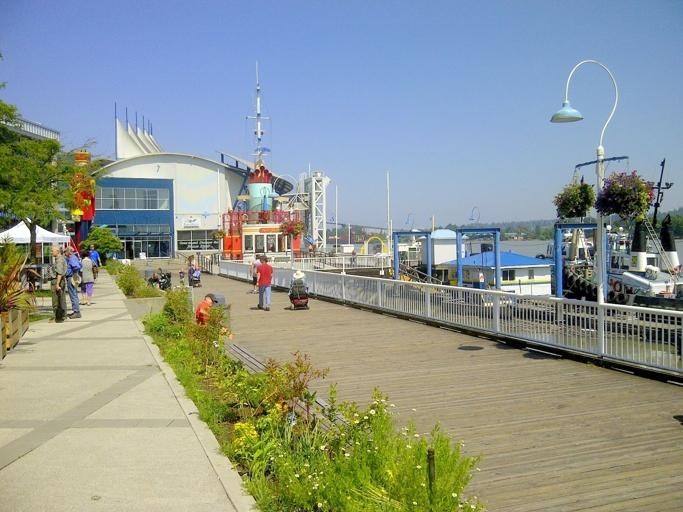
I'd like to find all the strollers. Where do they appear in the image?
[189,271,200,288]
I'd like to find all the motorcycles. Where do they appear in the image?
[155,267,172,290]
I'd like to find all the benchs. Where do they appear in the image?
[219,341,371,459]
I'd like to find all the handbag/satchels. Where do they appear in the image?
[55,290,64,320]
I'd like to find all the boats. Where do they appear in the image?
[543,156,683,346]
[440,290,515,320]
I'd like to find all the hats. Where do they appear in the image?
[71,274,82,288]
[293,270,305,279]
[206,294,218,303]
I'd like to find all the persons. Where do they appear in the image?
[255,256,273,311]
[63,246,82,320]
[349,250,357,267]
[78,250,96,306]
[250,254,261,294]
[196,293,218,325]
[187,263,195,281]
[87,243,102,280]
[48,245,68,323]
[192,267,200,279]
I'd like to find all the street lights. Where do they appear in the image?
[547,59,619,360]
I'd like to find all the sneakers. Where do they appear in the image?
[69,311,81,319]
[49,319,63,324]
[257,304,263,309]
[266,307,270,311]
[67,311,76,317]
